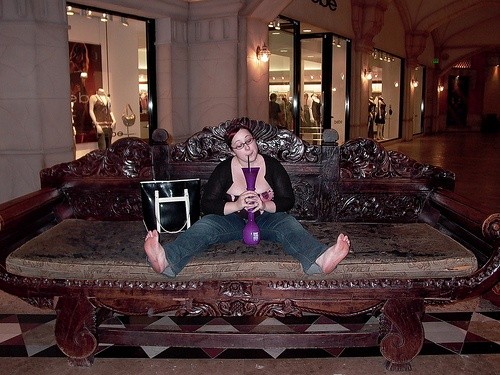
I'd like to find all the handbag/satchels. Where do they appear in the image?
[122,104,136,126]
[139,178,202,233]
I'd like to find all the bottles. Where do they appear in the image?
[242,167,260,246]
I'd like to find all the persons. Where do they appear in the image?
[368,96,386,140]
[89,88,116,151]
[269,94,281,127]
[144,124,350,278]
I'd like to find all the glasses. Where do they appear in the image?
[232,135,254,150]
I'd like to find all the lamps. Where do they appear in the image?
[67,6,75,16]
[333,36,342,48]
[439,82,445,92]
[412,77,419,88]
[362,68,373,80]
[108,15,114,23]
[371,48,395,63]
[268,19,281,30]
[256,43,272,63]
[100,14,107,22]
[121,17,129,27]
[87,11,93,19]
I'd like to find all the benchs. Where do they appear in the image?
[0,114,500,373]
